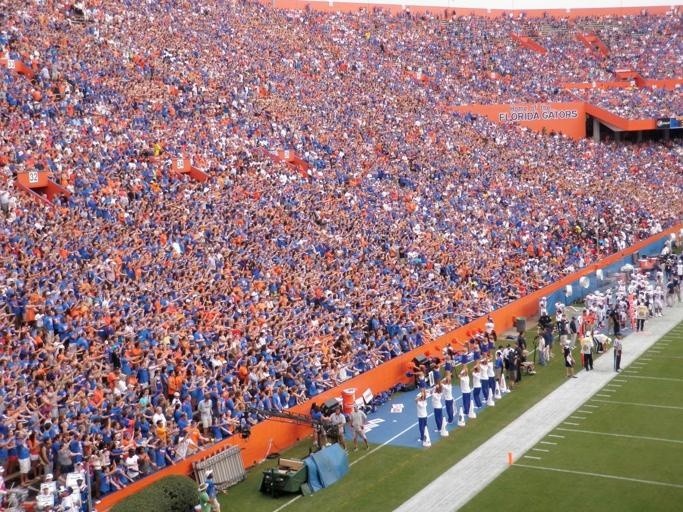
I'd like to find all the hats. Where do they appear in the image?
[506,344,511,348]
[206,469,213,477]
[616,333,623,335]
[197,482,209,492]
[335,405,342,409]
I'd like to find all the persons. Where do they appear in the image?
[0,0,682,512]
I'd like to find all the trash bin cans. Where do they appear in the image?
[320,388,355,415]
[515,318,525,332]
[412,354,426,366]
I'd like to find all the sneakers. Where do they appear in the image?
[344,448,348,451]
[366,446,370,451]
[463,413,469,416]
[648,313,663,318]
[615,368,621,372]
[482,397,487,404]
[354,448,358,452]
[568,374,578,378]
[314,441,318,445]
[474,406,481,408]
[433,429,440,432]
[326,442,332,446]
[621,327,628,330]
[586,366,593,370]
[418,438,422,442]
